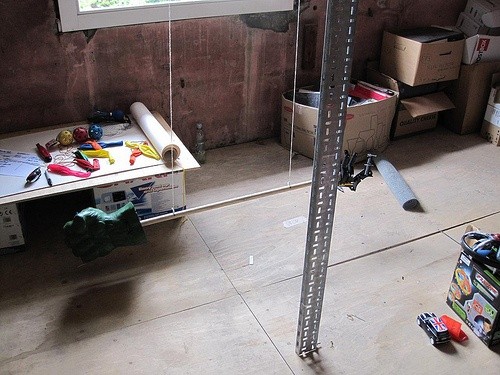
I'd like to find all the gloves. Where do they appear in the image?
[63,202,147,263]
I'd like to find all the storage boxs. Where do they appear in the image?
[0,204,26,248]
[92,172,188,222]
[446,233,500,348]
[277,0,500,163]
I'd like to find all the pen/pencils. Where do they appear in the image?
[45,170,52,186]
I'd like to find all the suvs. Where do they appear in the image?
[416,312,451,345]
